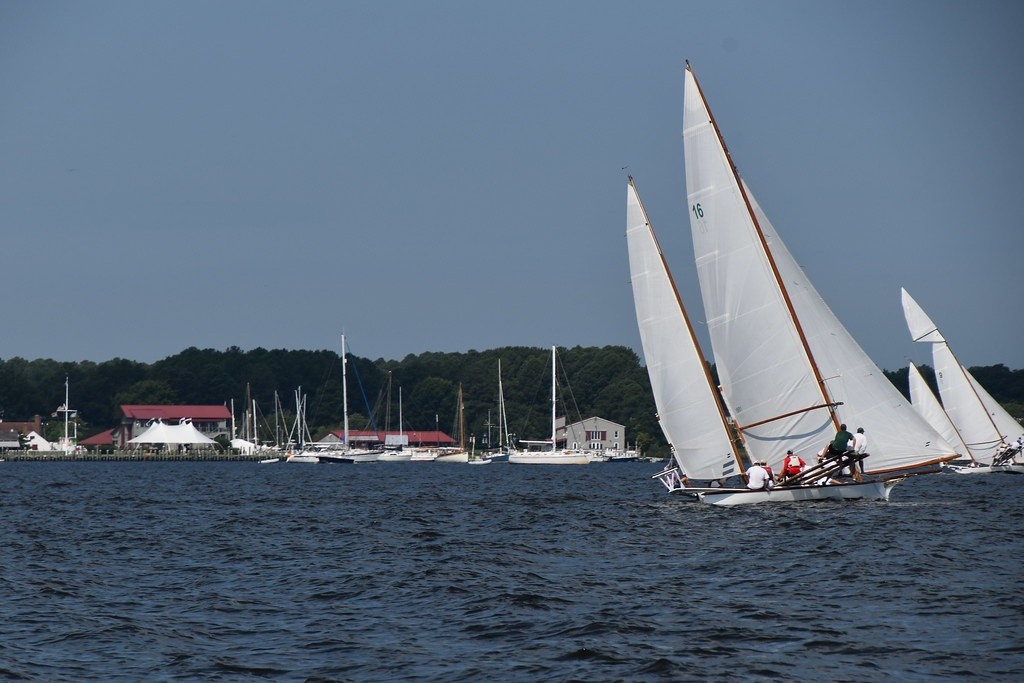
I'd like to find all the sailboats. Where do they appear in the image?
[625,57,964,508]
[231,328,665,465]
[898,286,1024,475]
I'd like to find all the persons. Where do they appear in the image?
[740,460,774,492]
[992,437,1022,461]
[777,450,806,480]
[707,479,723,488]
[849,428,868,473]
[817,440,841,485]
[830,424,856,458]
[664,465,688,485]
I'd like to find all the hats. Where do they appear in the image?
[787,450,793,454]
[761,460,767,463]
[753,460,760,464]
[857,428,865,433]
